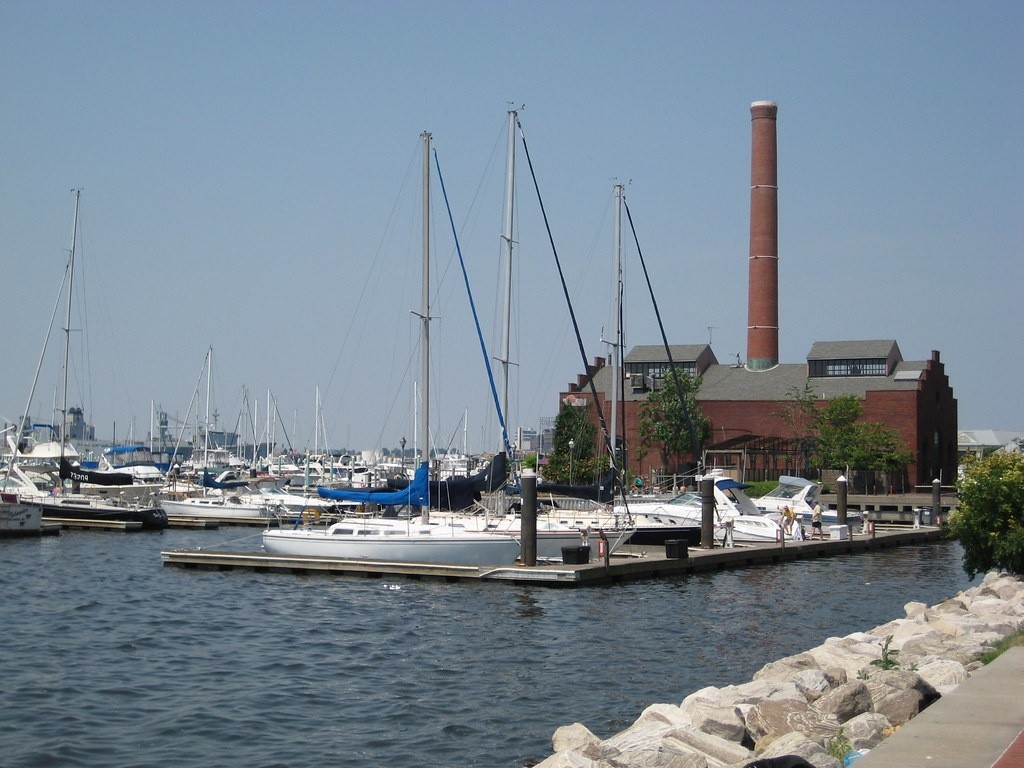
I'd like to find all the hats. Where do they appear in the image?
[811,500,818,504]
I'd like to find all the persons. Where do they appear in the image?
[779,505,796,535]
[632,475,686,495]
[809,500,824,540]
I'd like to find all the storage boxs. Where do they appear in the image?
[561,546,590,564]
[664,539,690,558]
[828,524,847,540]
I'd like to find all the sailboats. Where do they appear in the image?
[0,106,852,570]
